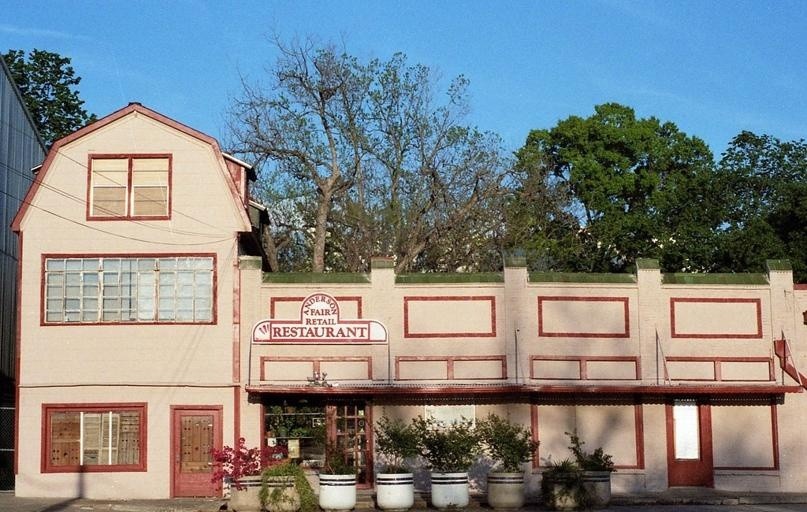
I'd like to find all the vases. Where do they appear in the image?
[230,475,262,512]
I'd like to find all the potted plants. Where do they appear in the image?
[473,410,540,511]
[310,418,362,512]
[370,415,433,511]
[563,429,618,510]
[260,412,323,511]
[542,457,583,510]
[413,414,493,510]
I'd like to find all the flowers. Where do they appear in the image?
[209,438,290,498]
